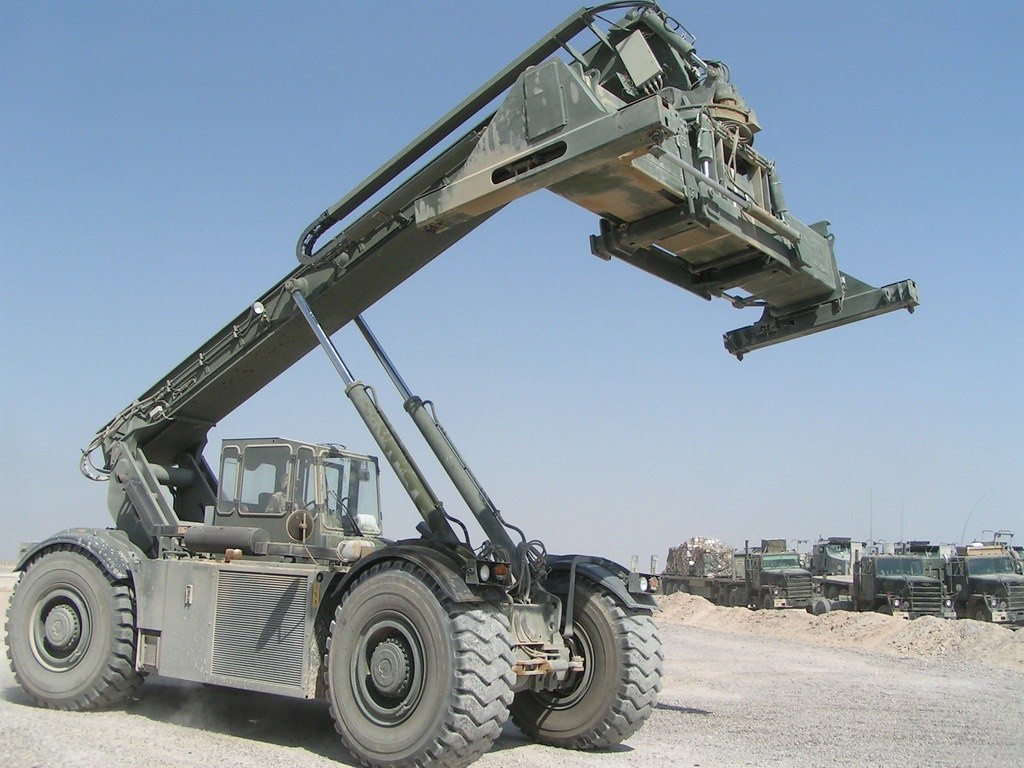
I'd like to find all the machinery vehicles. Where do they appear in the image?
[2,0,920,768]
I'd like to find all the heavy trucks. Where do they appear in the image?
[659,530,1024,631]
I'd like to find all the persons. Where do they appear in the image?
[265,473,309,512]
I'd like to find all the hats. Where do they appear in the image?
[280,473,300,490]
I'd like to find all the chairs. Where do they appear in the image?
[259,492,275,504]
